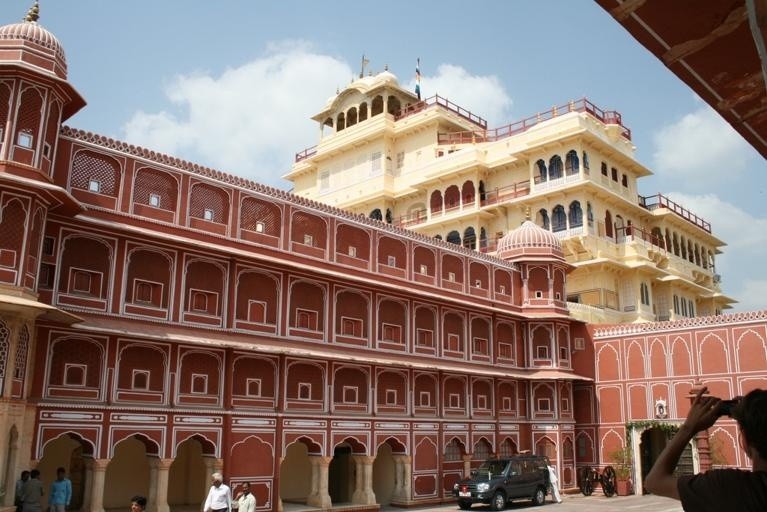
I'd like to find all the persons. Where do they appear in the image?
[21,469,45,512]
[13,470,30,512]
[201,472,232,512]
[231,481,256,512]
[642,384,767,512]
[130,495,147,512]
[47,466,73,512]
[546,464,562,504]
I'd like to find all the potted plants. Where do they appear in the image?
[608,443,634,497]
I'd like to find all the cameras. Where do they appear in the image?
[718,400,737,415]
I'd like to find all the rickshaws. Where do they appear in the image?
[577,461,617,498]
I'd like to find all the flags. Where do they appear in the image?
[361,55,370,68]
[414,59,420,94]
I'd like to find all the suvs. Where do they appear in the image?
[451,453,550,511]
